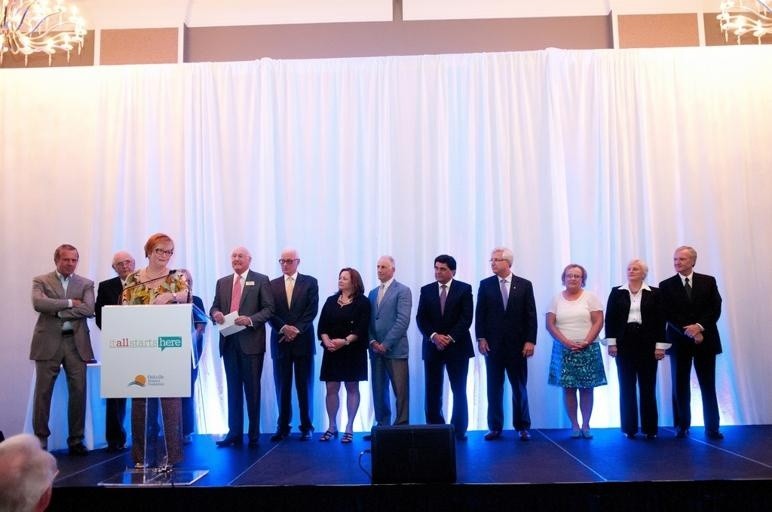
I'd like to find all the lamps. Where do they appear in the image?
[1,4,88,73]
[718,2,772,47]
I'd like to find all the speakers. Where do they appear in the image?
[372,426,456,484]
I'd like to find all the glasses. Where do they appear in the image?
[279,259,298,264]
[155,248,173,257]
[489,257,507,262]
[117,260,132,266]
[565,274,582,278]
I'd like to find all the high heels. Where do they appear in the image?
[42,444,48,451]
[320,429,338,442]
[341,430,353,444]
[645,432,657,442]
[625,432,635,440]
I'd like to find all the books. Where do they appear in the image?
[214,310,246,337]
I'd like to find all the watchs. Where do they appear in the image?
[172,292,178,303]
[344,337,349,345]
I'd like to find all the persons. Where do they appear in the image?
[209,245,276,450]
[604,258,672,439]
[317,267,371,444]
[173,269,208,445]
[29,244,95,457]
[121,233,188,470]
[416,254,475,441]
[659,246,724,440]
[545,264,608,439]
[94,250,135,453]
[0,434,60,512]
[268,246,319,441]
[475,246,537,440]
[362,254,412,441]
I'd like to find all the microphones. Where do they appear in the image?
[119,269,177,301]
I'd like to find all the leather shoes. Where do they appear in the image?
[216,437,244,446]
[571,425,582,438]
[104,444,124,456]
[364,434,377,442]
[67,442,93,458]
[300,428,314,441]
[706,430,723,440]
[272,429,289,441]
[584,425,593,439]
[674,426,690,438]
[455,433,468,442]
[484,429,502,441]
[247,437,260,449]
[518,429,532,441]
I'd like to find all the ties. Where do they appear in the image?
[230,277,242,313]
[440,285,447,316]
[286,277,293,311]
[500,279,508,310]
[684,278,692,303]
[378,284,385,304]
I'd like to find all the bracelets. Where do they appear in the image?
[583,340,589,346]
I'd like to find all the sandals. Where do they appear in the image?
[146,434,160,444]
[182,434,193,444]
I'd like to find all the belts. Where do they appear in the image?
[62,329,74,338]
